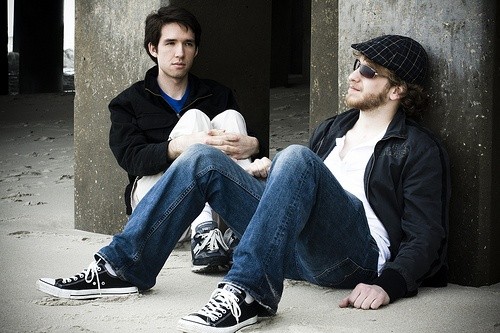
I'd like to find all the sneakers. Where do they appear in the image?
[224,227,239,265]
[178,283,258,333]
[190,221,229,274]
[36,259,138,300]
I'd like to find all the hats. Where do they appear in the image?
[350,34,428,86]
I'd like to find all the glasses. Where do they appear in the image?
[352,59,393,84]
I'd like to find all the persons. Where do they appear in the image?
[36,35,453,333]
[108,6,264,273]
